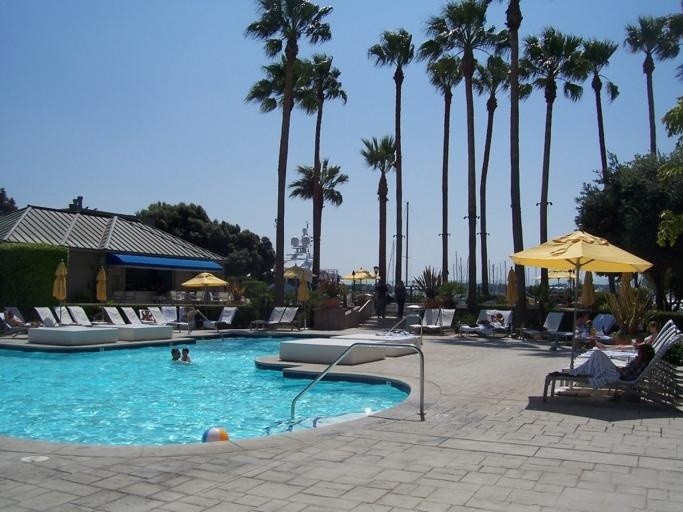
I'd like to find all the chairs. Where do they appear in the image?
[249,306,306,333]
[200,306,238,331]
[1,288,191,340]
[397,284,682,408]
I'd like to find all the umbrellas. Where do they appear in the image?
[534,269,577,287]
[181,269,229,315]
[95,264,106,320]
[298,270,311,330]
[343,267,377,293]
[508,224,653,396]
[52,259,69,325]
[505,267,518,311]
[284,263,310,293]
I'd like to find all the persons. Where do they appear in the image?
[479,314,504,332]
[171,348,180,359]
[174,306,197,323]
[3,308,41,326]
[568,310,658,381]
[179,349,191,363]
[140,310,154,321]
[394,279,408,319]
[377,277,389,318]
